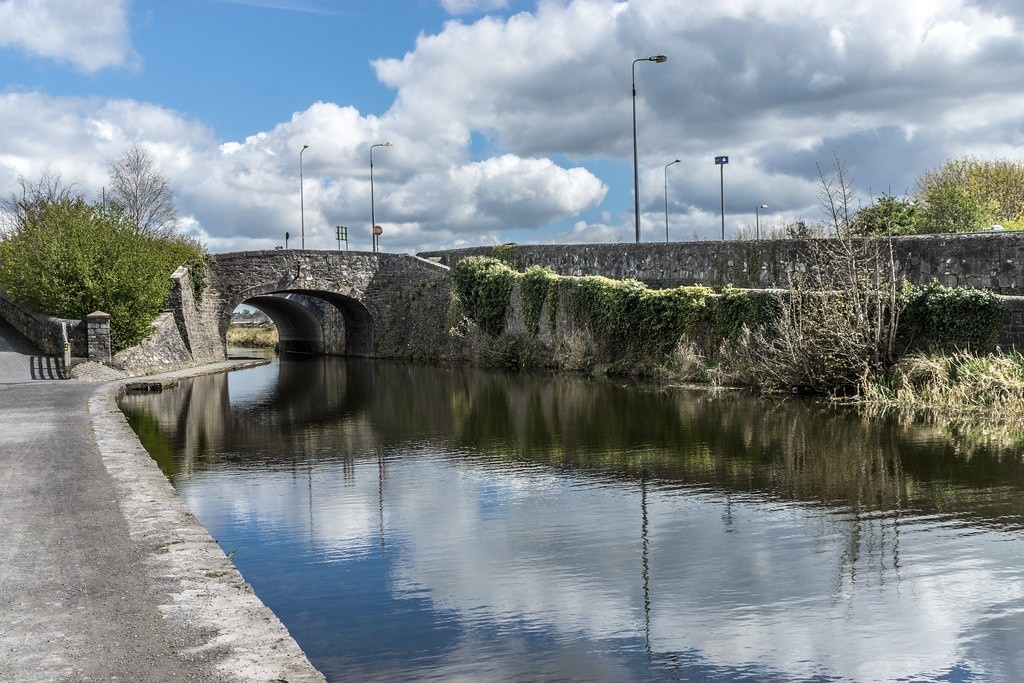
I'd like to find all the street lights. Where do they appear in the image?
[665,159,682,242]
[756,205,768,240]
[632,55,667,242]
[300,145,308,249]
[370,142,393,251]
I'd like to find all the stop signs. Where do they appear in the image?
[374,226,382,235]
